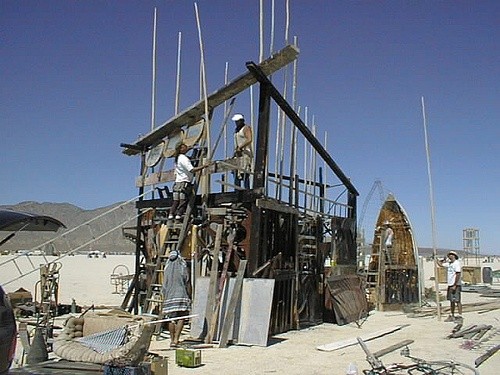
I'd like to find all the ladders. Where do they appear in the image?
[143,146,206,341]
[35,276,54,342]
[216,153,353,218]
[121,257,145,314]
[356,228,365,297]
[0,189,154,286]
[365,227,381,311]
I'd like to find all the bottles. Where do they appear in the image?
[72,301,76,312]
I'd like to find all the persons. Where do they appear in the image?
[166,141,211,224]
[381,220,394,265]
[162,249,192,348]
[435,250,463,322]
[231,114,255,191]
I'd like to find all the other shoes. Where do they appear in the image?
[167,218,174,224]
[170,341,187,348]
[444,315,455,322]
[455,315,462,320]
[174,218,182,224]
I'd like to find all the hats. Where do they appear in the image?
[447,251,459,259]
[231,114,244,120]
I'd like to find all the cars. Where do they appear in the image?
[0,208,67,375]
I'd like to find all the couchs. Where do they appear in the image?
[53,317,157,366]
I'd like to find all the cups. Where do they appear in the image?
[331,260,336,266]
